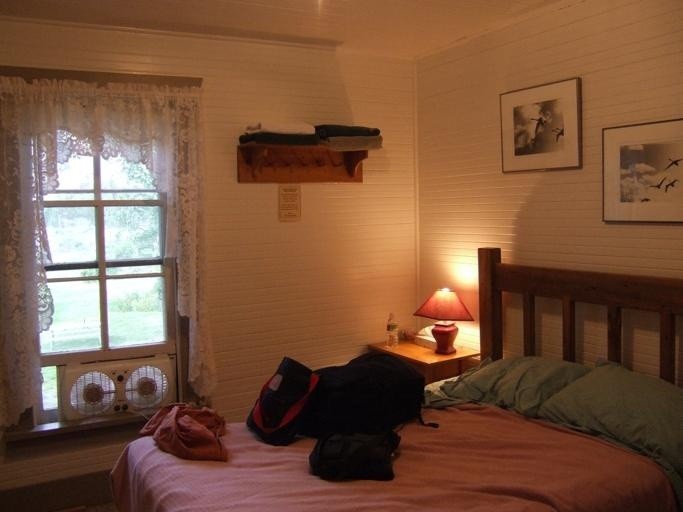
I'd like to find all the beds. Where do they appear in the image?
[127,245,681,512]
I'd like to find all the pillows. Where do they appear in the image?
[423,350,681,492]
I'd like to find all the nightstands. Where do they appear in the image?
[368,338,481,394]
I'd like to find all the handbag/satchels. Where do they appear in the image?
[297,351,426,440]
[245,356,320,447]
[308,429,401,482]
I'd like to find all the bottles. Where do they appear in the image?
[387,312,399,352]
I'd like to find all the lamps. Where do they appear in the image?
[412,286,474,355]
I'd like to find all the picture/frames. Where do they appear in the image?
[498,71,682,225]
